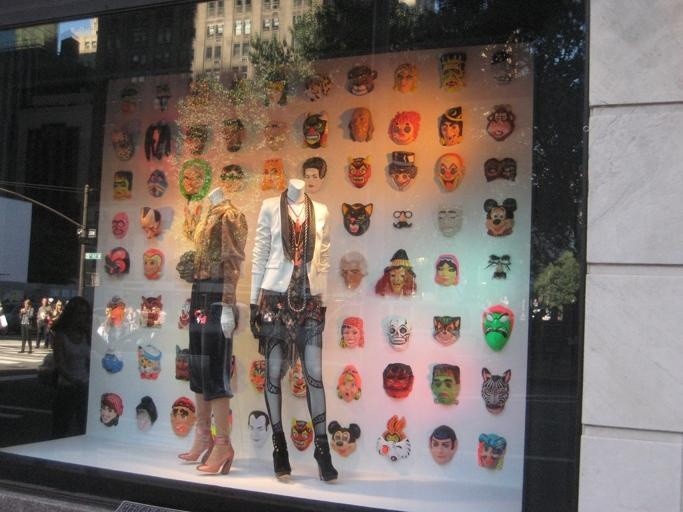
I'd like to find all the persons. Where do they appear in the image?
[0,298,64,353]
[249,180,339,482]
[49,296,91,439]
[179,187,247,474]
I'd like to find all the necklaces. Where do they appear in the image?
[286,192,309,313]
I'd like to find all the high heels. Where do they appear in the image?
[314,435,338,481]
[272,432,291,480]
[178,429,234,474]
